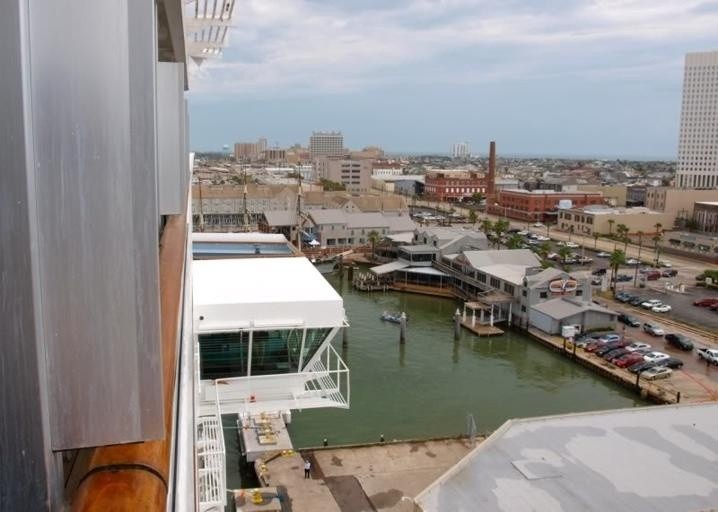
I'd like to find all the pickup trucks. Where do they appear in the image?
[696,347,718,366]
[663,332,694,350]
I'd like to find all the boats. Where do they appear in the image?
[381,312,409,325]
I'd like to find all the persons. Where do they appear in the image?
[303,459,311,479]
[622,324,626,335]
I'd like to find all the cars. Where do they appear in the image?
[641,300,663,310]
[613,352,642,368]
[612,273,634,282]
[591,276,602,285]
[657,358,685,369]
[596,251,611,258]
[709,302,718,311]
[622,314,641,327]
[486,226,593,265]
[575,333,634,362]
[592,267,606,275]
[624,341,652,352]
[641,366,672,380]
[643,351,670,364]
[614,290,646,307]
[693,297,718,306]
[412,209,465,225]
[624,258,677,280]
[618,312,625,322]
[651,304,672,313]
[534,222,544,227]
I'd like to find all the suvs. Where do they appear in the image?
[643,322,663,336]
